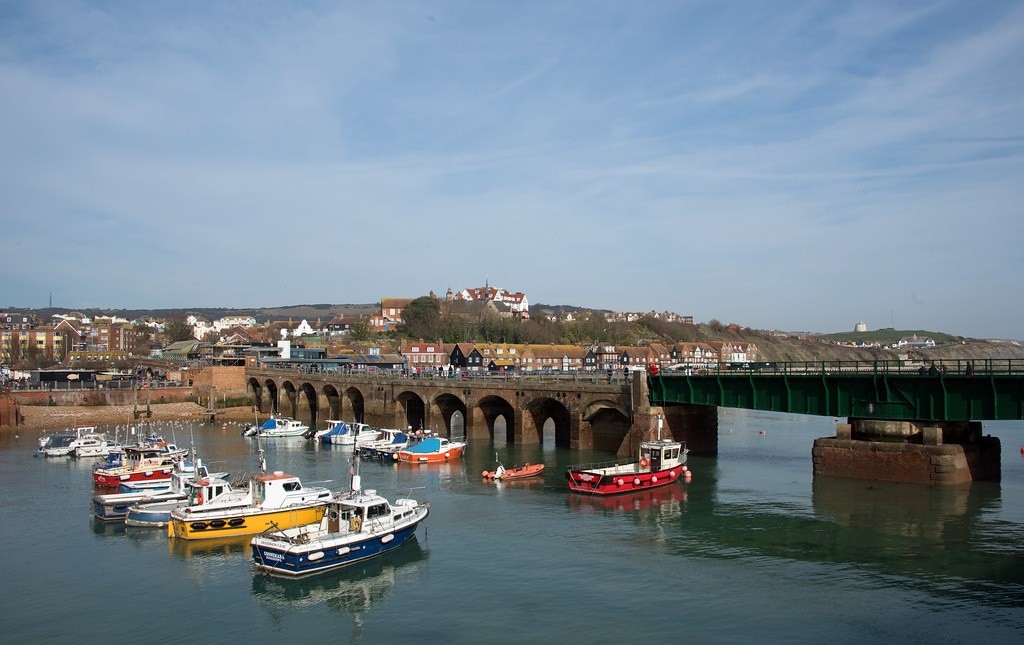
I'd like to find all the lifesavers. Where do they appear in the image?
[198,493,203,505]
[351,518,361,531]
[153,442,168,446]
[200,482,209,486]
[640,457,649,469]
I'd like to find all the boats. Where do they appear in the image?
[118,423,231,493]
[314,420,353,443]
[483,452,546,481]
[166,406,342,540]
[355,426,439,460]
[125,459,250,527]
[566,413,689,495]
[330,422,382,445]
[37,425,189,487]
[396,432,469,464]
[242,399,310,436]
[251,430,429,575]
[92,472,196,522]
